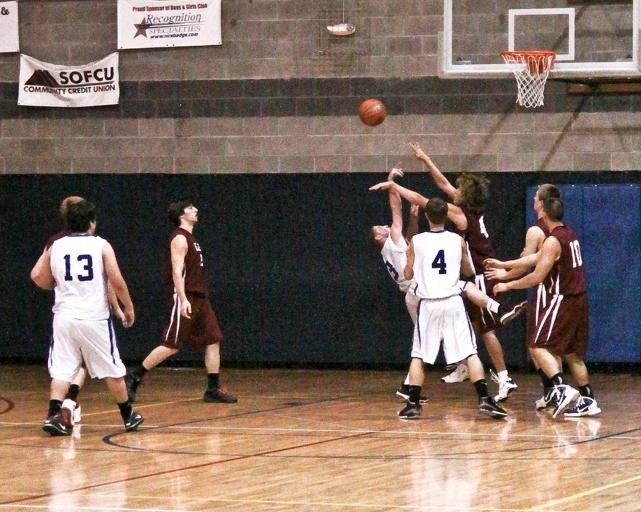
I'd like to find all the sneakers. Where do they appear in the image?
[440,363,470,384]
[204,384,237,403]
[395,402,420,419]
[496,300,528,326]
[42,404,143,436]
[395,381,429,403]
[123,368,145,402]
[478,395,508,419]
[489,369,519,403]
[535,384,600,419]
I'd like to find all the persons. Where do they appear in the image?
[121,194,238,404]
[482,196,604,420]
[40,194,129,427]
[30,200,145,436]
[397,196,512,422]
[482,182,564,410]
[366,139,520,406]
[359,160,529,406]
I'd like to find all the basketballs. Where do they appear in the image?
[358,97,387,126]
